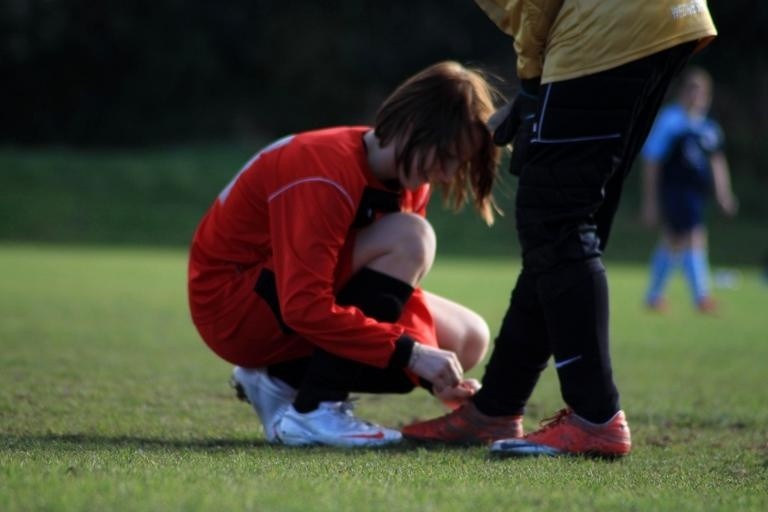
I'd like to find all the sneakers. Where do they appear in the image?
[229,366,406,449]
[492,409,632,458]
[402,400,525,446]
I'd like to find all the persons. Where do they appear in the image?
[401,0,718,458]
[638,71,736,315]
[188,61,515,449]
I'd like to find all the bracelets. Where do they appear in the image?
[409,343,422,369]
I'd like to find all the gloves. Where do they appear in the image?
[487,74,542,176]
